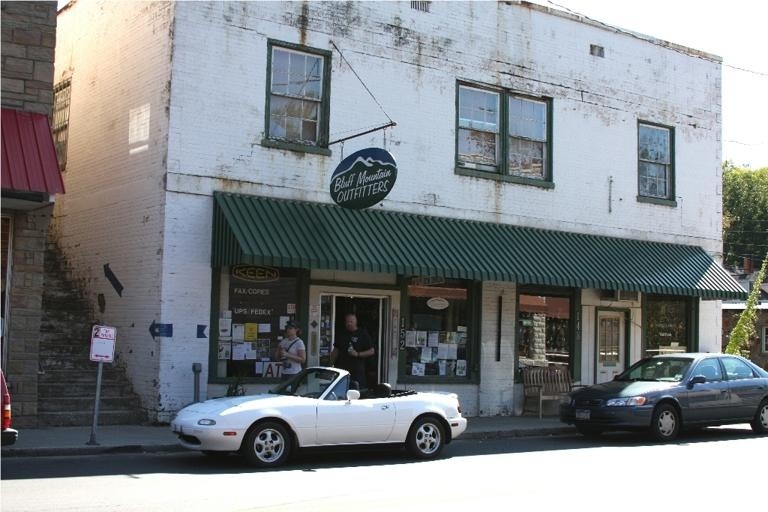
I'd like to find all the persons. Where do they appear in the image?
[330,311,376,390]
[272,320,305,386]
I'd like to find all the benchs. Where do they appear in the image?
[520,366,590,419]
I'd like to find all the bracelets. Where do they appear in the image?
[286,352,289,357]
[357,350,360,359]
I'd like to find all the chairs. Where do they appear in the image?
[349,381,360,391]
[652,361,671,378]
[729,367,751,379]
[700,366,717,379]
[365,383,392,398]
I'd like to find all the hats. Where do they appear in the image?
[286,320,302,329]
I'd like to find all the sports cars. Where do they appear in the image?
[169,364,468,467]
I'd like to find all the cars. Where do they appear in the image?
[558,353,768,439]
